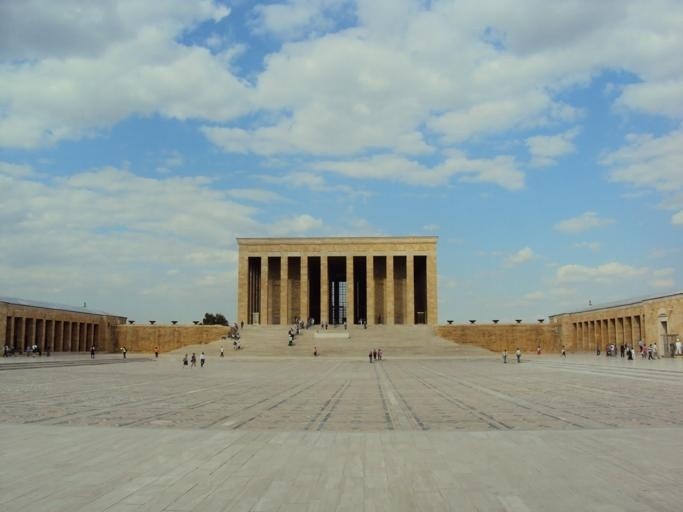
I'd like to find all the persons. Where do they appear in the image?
[560,345,566,359]
[319,321,324,331]
[89,345,95,359]
[367,348,382,364]
[595,339,660,361]
[668,341,675,358]
[675,338,682,355]
[343,322,347,330]
[312,347,317,356]
[228,320,244,351]
[287,315,315,346]
[190,352,196,368]
[219,346,223,357]
[536,345,541,355]
[325,322,328,329]
[153,347,159,358]
[514,347,521,363]
[501,349,507,364]
[181,353,188,368]
[0,343,51,358]
[119,346,126,360]
[198,352,206,367]
